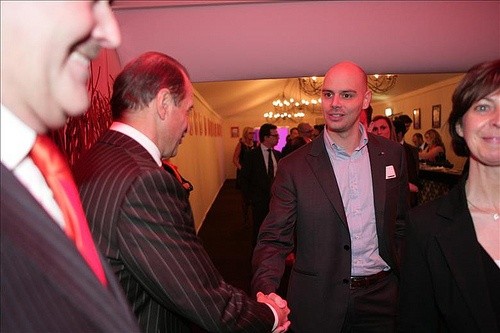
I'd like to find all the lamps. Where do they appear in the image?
[264,73,397,123]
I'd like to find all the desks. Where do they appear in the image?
[418,165,461,205]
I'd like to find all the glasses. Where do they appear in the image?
[270,134,279,138]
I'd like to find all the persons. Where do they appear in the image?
[242,124,285,247]
[398,58,500,333]
[370,114,454,206]
[233,127,256,189]
[0,1,144,333]
[72,53,288,333]
[282,122,325,157]
[253,60,413,333]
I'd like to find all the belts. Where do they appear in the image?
[349,269,394,289]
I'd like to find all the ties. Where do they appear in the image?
[267,149,274,178]
[29,137,106,285]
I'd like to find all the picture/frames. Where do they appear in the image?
[432,104,441,128]
[413,108,421,129]
